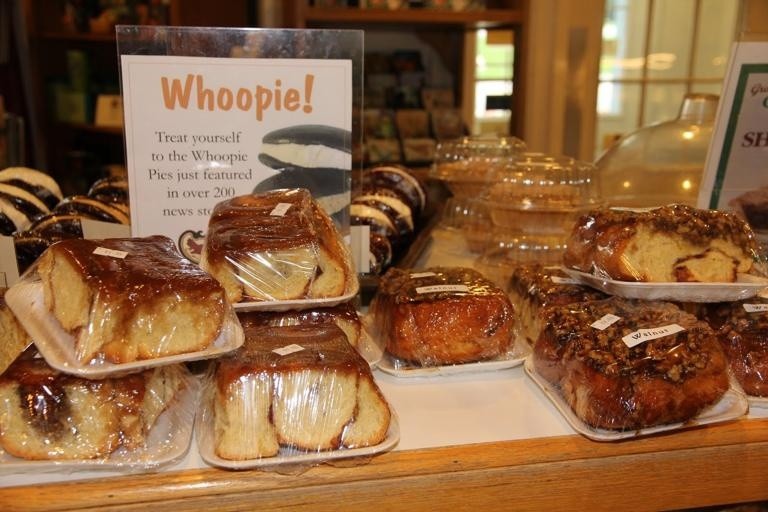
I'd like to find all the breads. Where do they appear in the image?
[0,152,435,470]
[429,150,768,443]
[364,262,522,376]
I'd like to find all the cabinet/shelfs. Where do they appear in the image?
[23,1,181,197]
[285,1,531,177]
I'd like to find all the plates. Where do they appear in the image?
[1,266,767,474]
